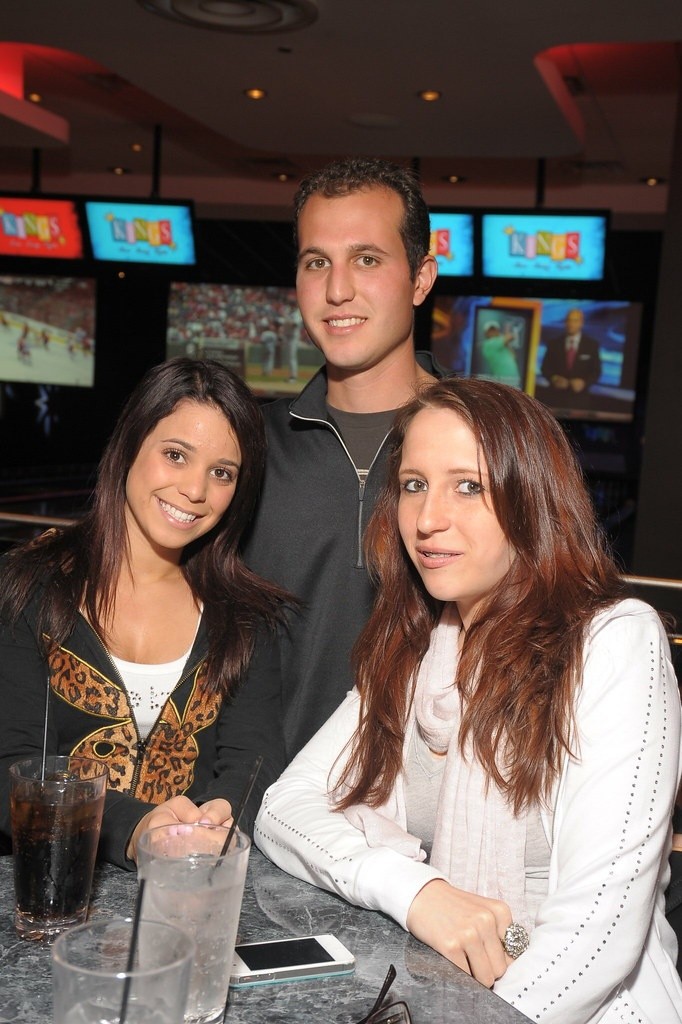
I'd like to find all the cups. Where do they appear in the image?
[135,823,252,1024]
[51,918,194,1024]
[8,755,111,937]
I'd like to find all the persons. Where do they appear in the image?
[540,309,602,393]
[252,378,682,1022]
[481,318,519,388]
[238,158,455,765]
[0,283,95,362]
[0,354,313,873]
[167,283,313,382]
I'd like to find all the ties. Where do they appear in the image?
[567,337,576,368]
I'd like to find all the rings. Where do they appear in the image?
[500,921,530,959]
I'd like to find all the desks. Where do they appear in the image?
[0,842,536,1024]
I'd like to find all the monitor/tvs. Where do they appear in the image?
[0,191,648,426]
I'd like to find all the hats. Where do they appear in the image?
[484,321,498,335]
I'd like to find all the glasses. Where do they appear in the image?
[355,964,411,1024]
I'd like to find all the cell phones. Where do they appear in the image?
[230,933,355,983]
